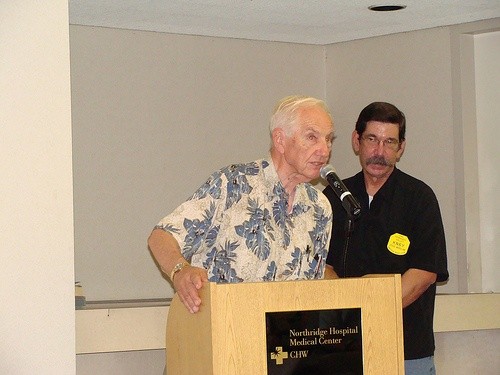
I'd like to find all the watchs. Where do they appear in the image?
[170,262,190,281]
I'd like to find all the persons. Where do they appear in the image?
[323,101,450,375]
[147,94,338,375]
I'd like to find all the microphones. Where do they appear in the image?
[320,164,361,218]
